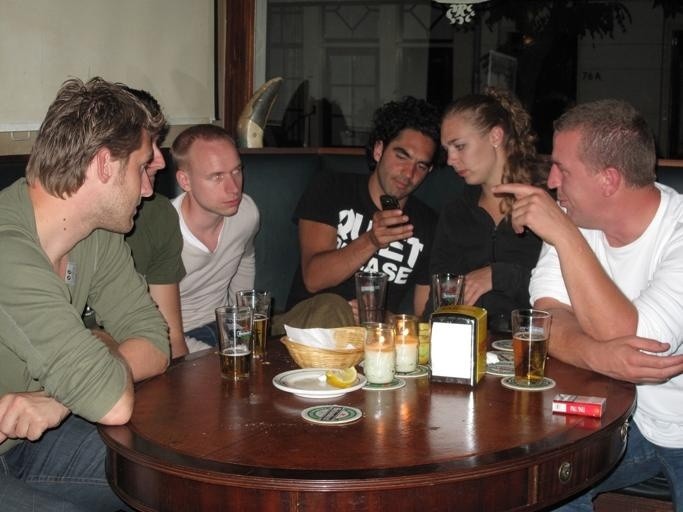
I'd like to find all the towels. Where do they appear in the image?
[284,322,357,350]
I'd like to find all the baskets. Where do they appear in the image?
[281,325,367,369]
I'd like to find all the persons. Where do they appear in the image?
[0,78,170,462]
[270,95,449,341]
[168,124,260,348]
[490,99,683,512]
[81,82,189,360]
[423,83,539,329]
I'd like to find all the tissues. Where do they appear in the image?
[427,304,488,386]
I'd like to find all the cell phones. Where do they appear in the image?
[381,193,402,229]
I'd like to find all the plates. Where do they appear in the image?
[273,367,366,400]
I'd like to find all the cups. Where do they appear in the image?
[237,288,272,359]
[364,322,397,385]
[511,309,550,387]
[355,270,390,326]
[432,273,465,309]
[216,305,255,382]
[392,315,419,373]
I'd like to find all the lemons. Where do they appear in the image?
[326,366,357,386]
